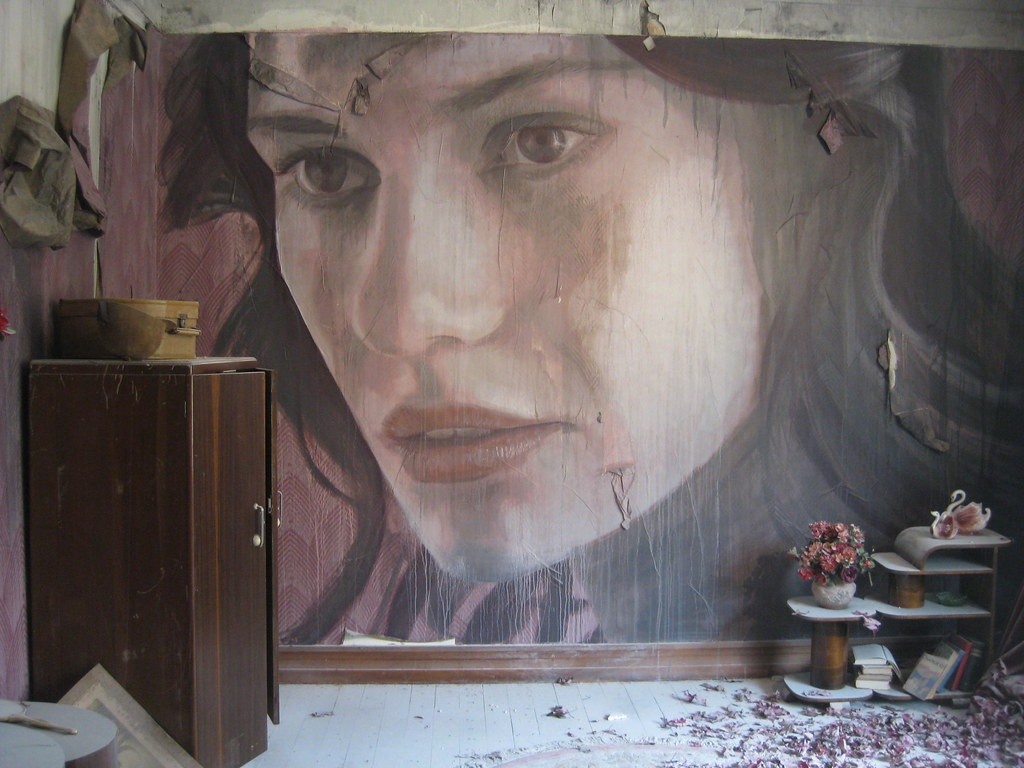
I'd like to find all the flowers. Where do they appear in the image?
[786,520,876,587]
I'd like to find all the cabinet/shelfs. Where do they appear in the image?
[784,528,1013,712]
[17,353,281,768]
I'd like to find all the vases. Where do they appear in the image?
[811,581,857,610]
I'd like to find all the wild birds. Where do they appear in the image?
[930,489,992,540]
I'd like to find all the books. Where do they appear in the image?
[903,635,986,702]
[850,644,905,690]
